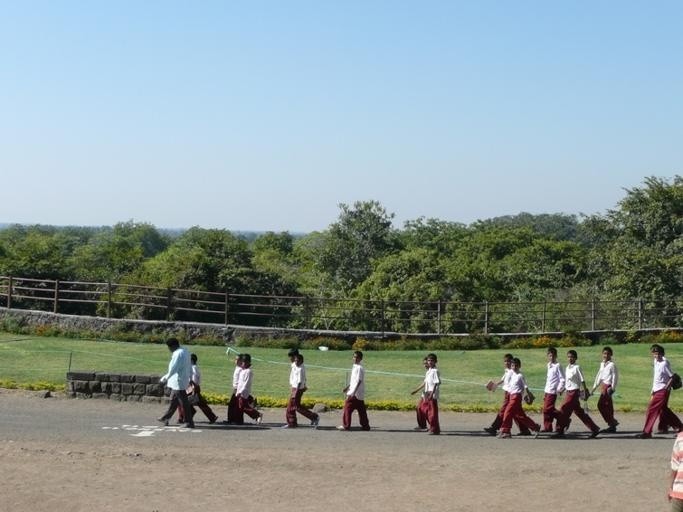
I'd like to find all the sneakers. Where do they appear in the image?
[588,429,616,437]
[517,424,541,438]
[635,433,651,439]
[428,430,440,434]
[183,408,196,421]
[414,426,428,431]
[336,426,346,431]
[484,426,511,438]
[223,420,236,425]
[209,416,218,424]
[250,414,263,425]
[176,419,194,428]
[539,430,564,435]
[284,424,297,428]
[157,417,169,425]
[361,426,370,431]
[311,416,320,425]
[655,424,683,434]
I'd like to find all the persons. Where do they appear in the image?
[666,431,683,512]
[556,350,600,439]
[177,354,218,424]
[336,351,370,431]
[425,353,440,434]
[157,338,196,428]
[500,358,541,439]
[288,348,298,368]
[484,354,531,435]
[589,347,619,432]
[235,354,262,425]
[282,354,320,428]
[411,357,429,429]
[539,347,571,433]
[636,346,683,439]
[650,344,672,434]
[222,355,242,425]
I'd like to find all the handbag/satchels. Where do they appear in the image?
[185,384,200,404]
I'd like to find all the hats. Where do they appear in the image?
[242,354,250,361]
[652,347,664,352]
